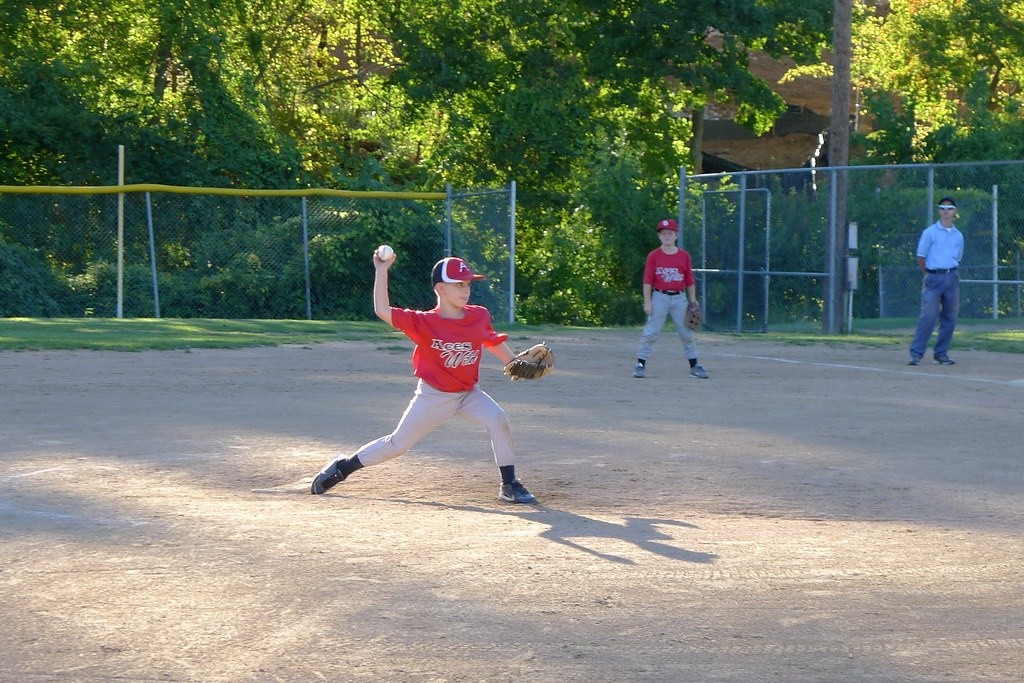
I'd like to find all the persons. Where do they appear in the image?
[310,249,554,504]
[909,196,965,365]
[634,220,709,380]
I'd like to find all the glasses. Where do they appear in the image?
[939,204,955,210]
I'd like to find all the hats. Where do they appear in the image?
[939,196,958,207]
[657,220,678,232]
[431,257,485,287]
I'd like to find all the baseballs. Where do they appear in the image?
[378,245,395,261]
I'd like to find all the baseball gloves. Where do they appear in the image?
[503,344,555,383]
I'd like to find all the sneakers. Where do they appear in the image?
[689,363,709,379]
[906,356,923,365]
[933,355,954,365]
[634,362,646,378]
[310,455,346,494]
[499,479,537,506]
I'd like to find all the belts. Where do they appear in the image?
[927,267,957,274]
[655,288,684,295]
